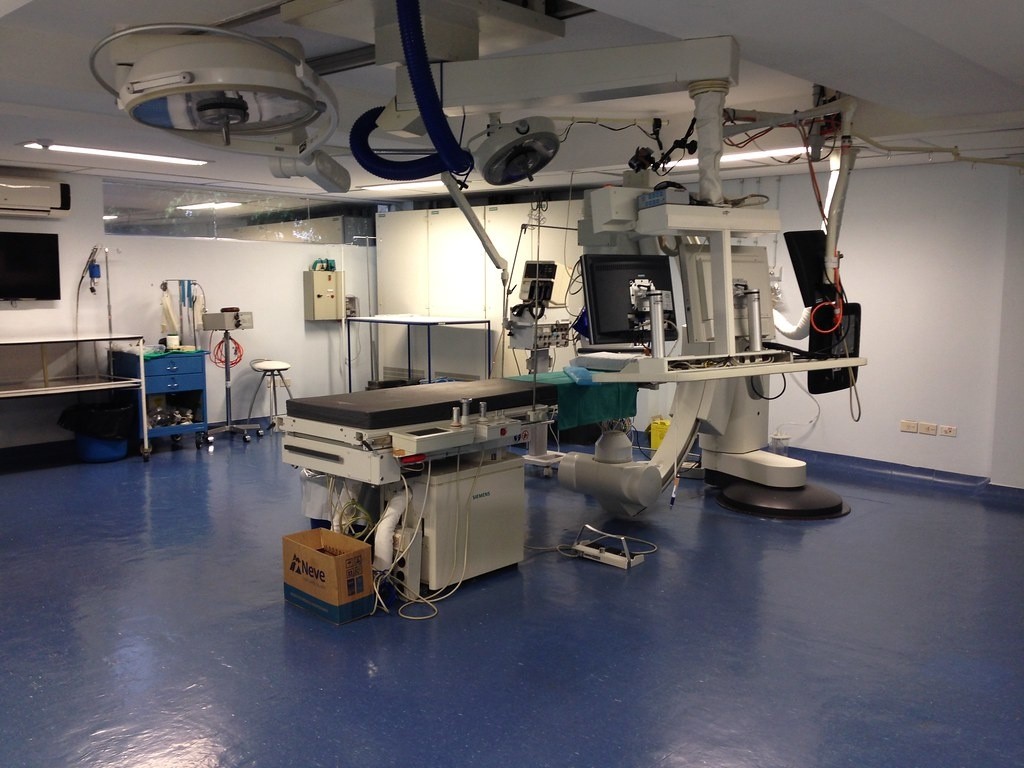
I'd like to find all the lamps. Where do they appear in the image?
[471,114,561,187]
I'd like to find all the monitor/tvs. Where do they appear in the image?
[783,230,843,307]
[580,252,679,346]
[678,243,776,345]
[0,232,60,301]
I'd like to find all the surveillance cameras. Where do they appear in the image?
[628,148,654,170]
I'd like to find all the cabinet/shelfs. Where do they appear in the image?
[344,312,492,393]
[0,331,154,463]
[105,346,211,455]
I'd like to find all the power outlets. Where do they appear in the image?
[939,425,957,437]
[918,422,938,436]
[900,419,918,433]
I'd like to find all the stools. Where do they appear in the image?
[241,360,296,443]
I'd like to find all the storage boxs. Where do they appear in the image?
[54,399,139,464]
[282,527,377,626]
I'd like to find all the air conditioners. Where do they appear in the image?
[0,174,72,220]
